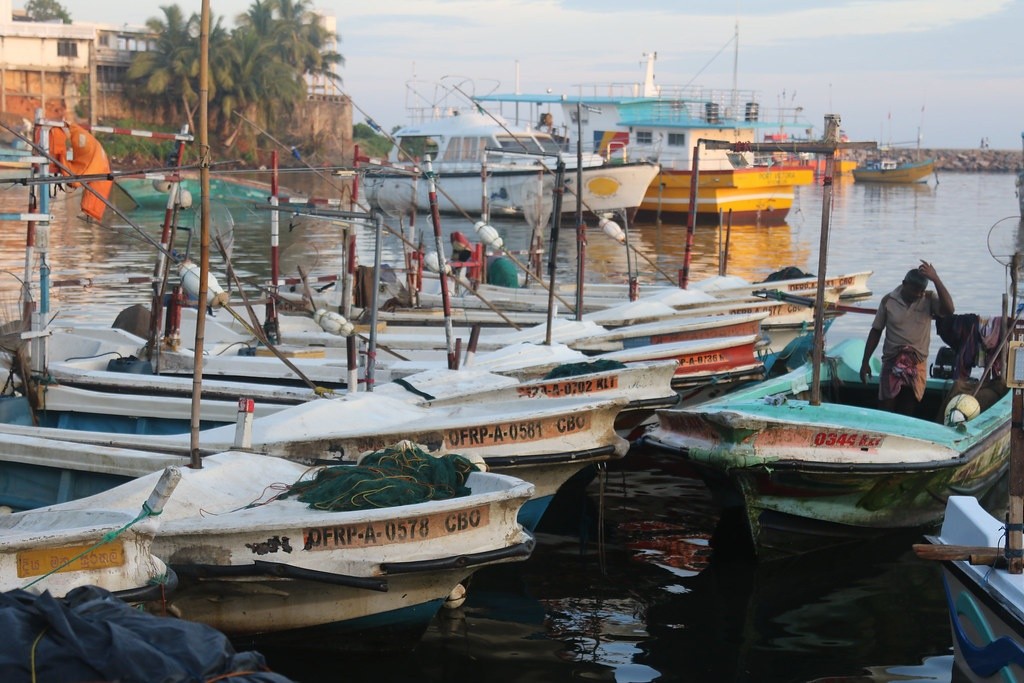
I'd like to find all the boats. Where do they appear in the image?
[853,132,939,184]
[0,142,1024,682]
[354,71,663,233]
[469,50,815,227]
[760,91,830,183]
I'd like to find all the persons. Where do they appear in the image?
[859,259,954,414]
